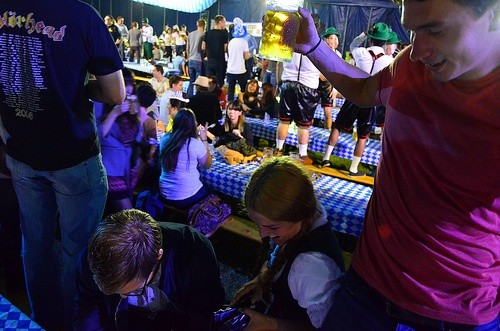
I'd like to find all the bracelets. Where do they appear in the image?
[303,36,322,56]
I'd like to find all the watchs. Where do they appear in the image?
[201,137,207,141]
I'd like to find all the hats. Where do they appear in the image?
[321,27,341,38]
[142,17,149,23]
[166,25,170,31]
[179,31,185,35]
[173,24,179,31]
[192,75,212,87]
[367,23,392,40]
[387,31,401,44]
[231,24,247,38]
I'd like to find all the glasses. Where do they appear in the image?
[124,277,149,296]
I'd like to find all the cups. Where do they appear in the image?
[127,97,139,114]
[259,0,305,63]
[289,152,299,160]
[273,118,278,127]
[208,144,214,161]
[235,85,240,95]
[353,127,358,141]
[263,147,273,160]
[224,85,228,94]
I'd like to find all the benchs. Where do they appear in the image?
[264,154,374,186]
[220,216,352,274]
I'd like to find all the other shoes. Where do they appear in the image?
[348,171,366,178]
[274,149,284,156]
[322,160,331,167]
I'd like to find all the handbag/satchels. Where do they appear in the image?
[185,193,231,235]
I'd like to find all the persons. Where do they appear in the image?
[92,67,143,210]
[103,14,159,64]
[316,27,342,131]
[229,163,344,331]
[156,24,189,64]
[273,14,326,160]
[187,15,262,103]
[258,15,283,85]
[354,31,401,141]
[213,101,253,147]
[233,80,279,119]
[73,208,225,331]
[320,23,393,176]
[126,65,190,148]
[183,72,222,140]
[278,0,500,331]
[159,108,212,210]
[0,0,126,331]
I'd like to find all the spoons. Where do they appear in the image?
[207,124,216,129]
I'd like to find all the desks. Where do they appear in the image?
[314,104,359,127]
[219,115,383,167]
[197,151,366,239]
[123,62,178,77]
